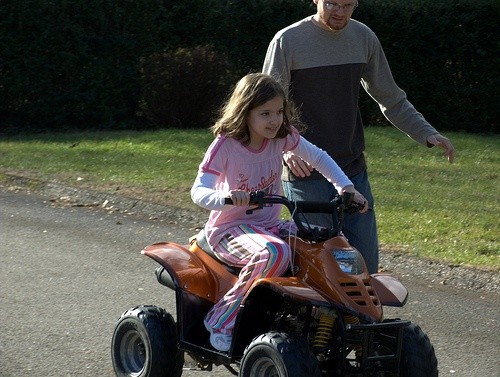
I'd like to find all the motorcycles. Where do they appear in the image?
[108,189,439,376]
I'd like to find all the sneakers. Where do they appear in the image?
[204,320,232,351]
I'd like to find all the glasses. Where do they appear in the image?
[322,0,359,11]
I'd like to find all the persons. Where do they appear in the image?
[255,0,459,279]
[188,71,369,353]
